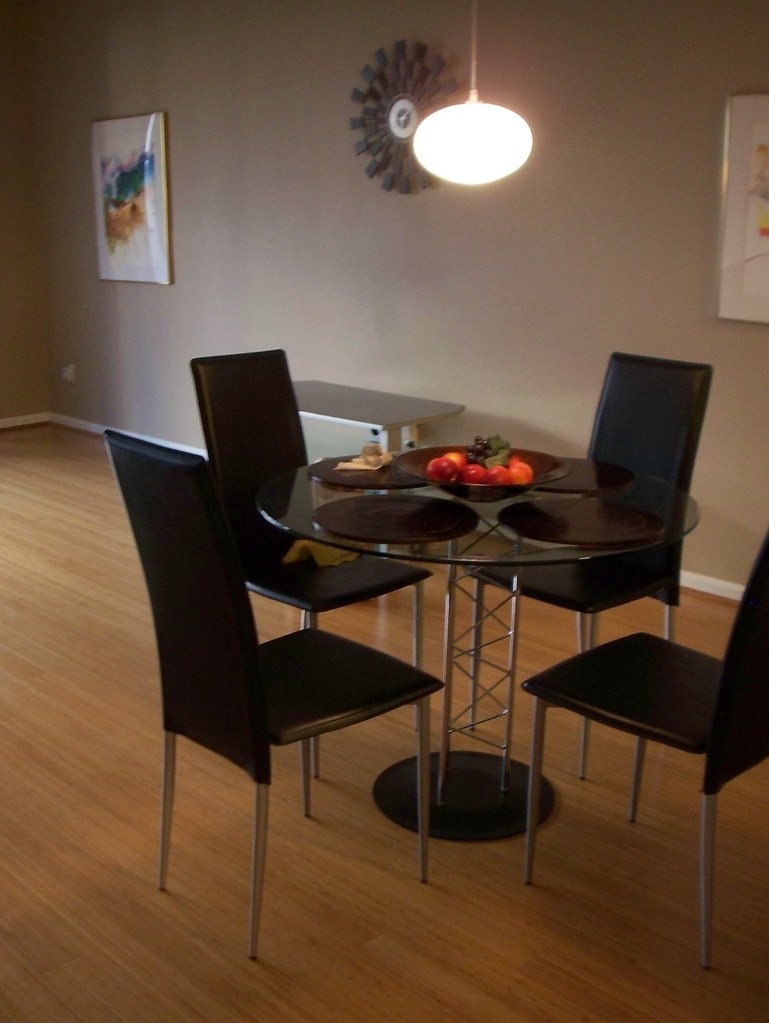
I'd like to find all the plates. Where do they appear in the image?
[311,494,479,542]
[497,499,666,546]
[531,457,636,494]
[307,453,429,489]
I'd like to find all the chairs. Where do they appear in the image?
[102,349,769,968]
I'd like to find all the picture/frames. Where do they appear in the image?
[716,91,769,324]
[91,112,172,285]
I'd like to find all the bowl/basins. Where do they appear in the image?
[393,444,573,502]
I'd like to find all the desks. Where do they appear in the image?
[255,451,701,841]
[291,380,465,454]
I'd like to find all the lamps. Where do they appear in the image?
[412,1,535,186]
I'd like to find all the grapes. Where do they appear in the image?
[465,435,495,464]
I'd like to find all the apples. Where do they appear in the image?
[425,452,533,486]
